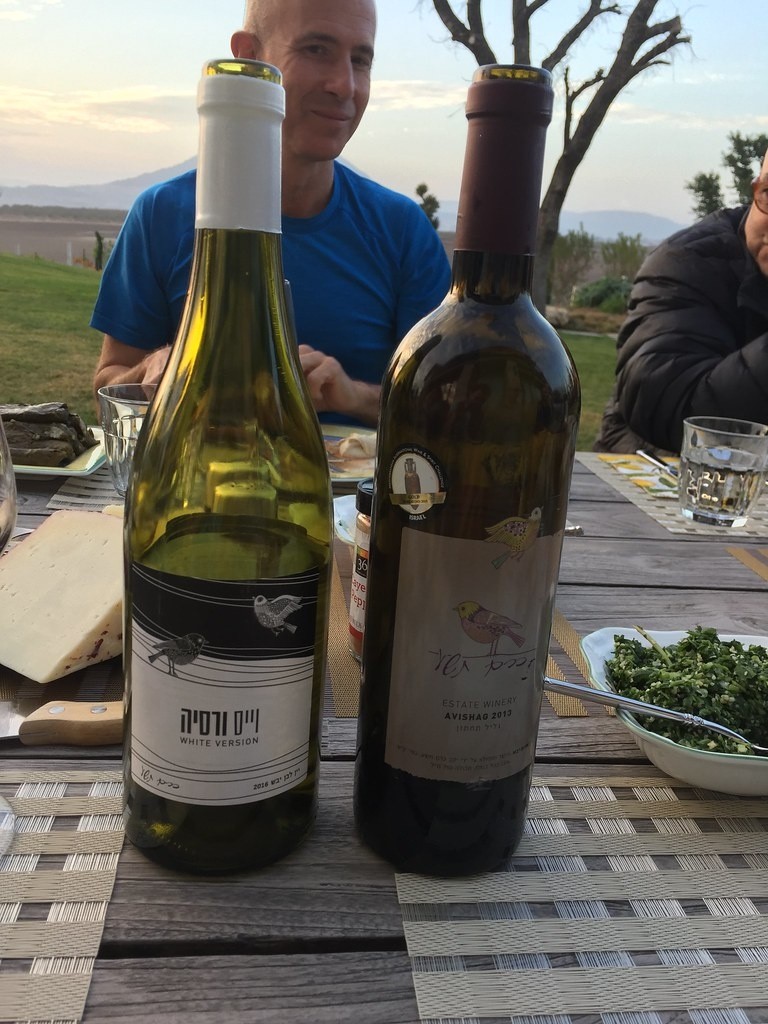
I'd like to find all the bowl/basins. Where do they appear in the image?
[331,495,355,563]
[578,624,768,797]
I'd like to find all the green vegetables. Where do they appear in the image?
[605,621,768,758]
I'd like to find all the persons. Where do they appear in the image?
[94,2,453,433]
[588,146,768,456]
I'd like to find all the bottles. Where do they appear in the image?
[351,64,583,877]
[124,64,335,878]
[348,477,374,662]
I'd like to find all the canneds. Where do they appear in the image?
[347,477,373,661]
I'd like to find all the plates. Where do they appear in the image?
[319,423,377,482]
[12,428,107,481]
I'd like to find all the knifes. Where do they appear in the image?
[0,698,123,747]
[637,448,677,482]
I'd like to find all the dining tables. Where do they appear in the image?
[0,448,767,1023]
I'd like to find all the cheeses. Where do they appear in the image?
[0,503,136,683]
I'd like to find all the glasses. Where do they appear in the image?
[751,177,768,214]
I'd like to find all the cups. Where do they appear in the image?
[98,383,158,498]
[0,418,17,556]
[677,416,768,528]
